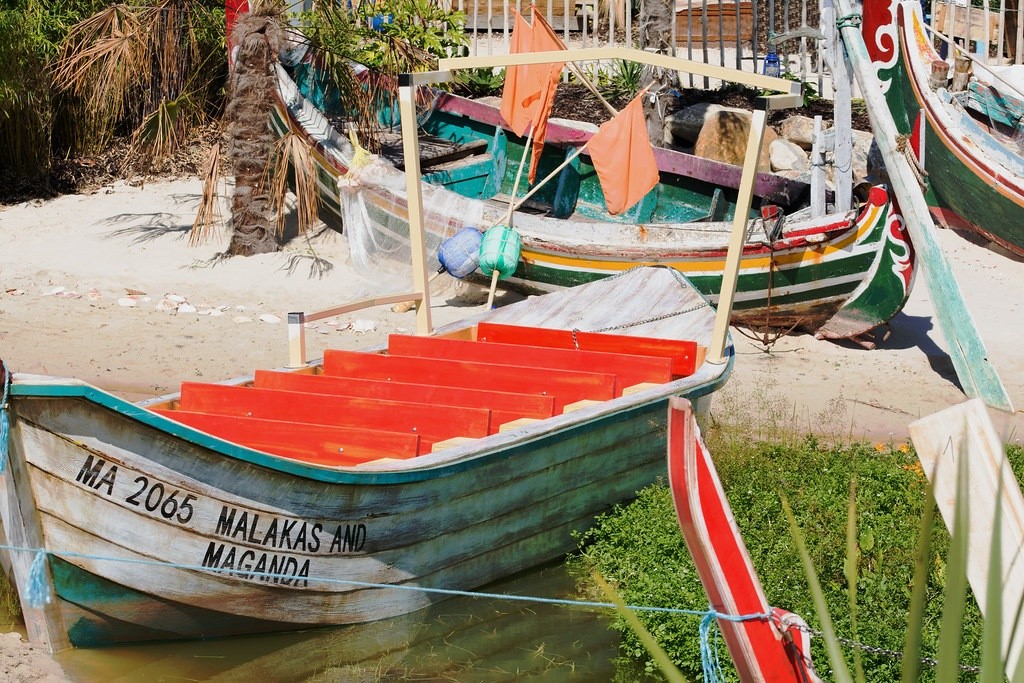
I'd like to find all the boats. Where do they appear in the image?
[223,0,928,341]
[5,42,810,638]
[858,1,1024,261]
